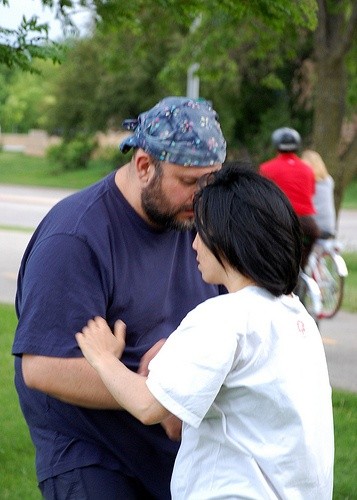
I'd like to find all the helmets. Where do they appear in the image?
[271,127,301,152]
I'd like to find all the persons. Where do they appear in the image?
[75,166,336,500]
[259,126,341,297]
[11,95,226,500]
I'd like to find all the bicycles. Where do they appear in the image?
[291,229,349,329]
